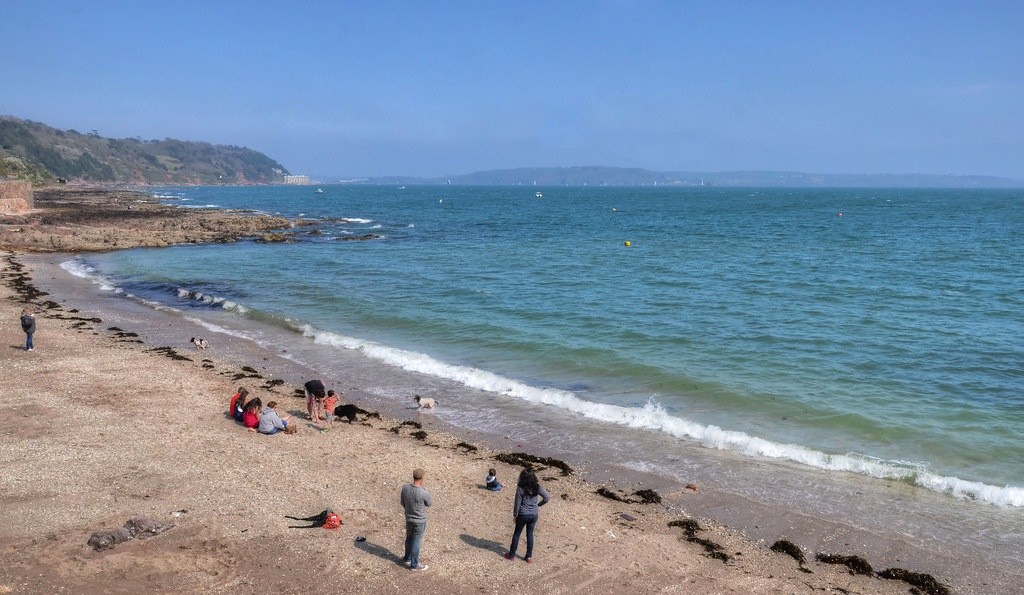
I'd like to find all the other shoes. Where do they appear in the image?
[410,562,427,571]
[525,556,532,563]
[404,560,411,568]
[504,552,514,561]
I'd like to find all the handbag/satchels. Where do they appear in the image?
[283,425,297,434]
[315,506,340,529]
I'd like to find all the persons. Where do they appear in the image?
[502,467,550,563]
[399,468,432,572]
[21,307,36,351]
[485,468,503,491]
[242,397,293,436]
[230,386,246,418]
[304,378,340,432]
[235,391,251,422]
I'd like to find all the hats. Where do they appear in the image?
[413,468,425,478]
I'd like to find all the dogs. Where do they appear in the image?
[413,395,438,410]
[189,337,209,350]
